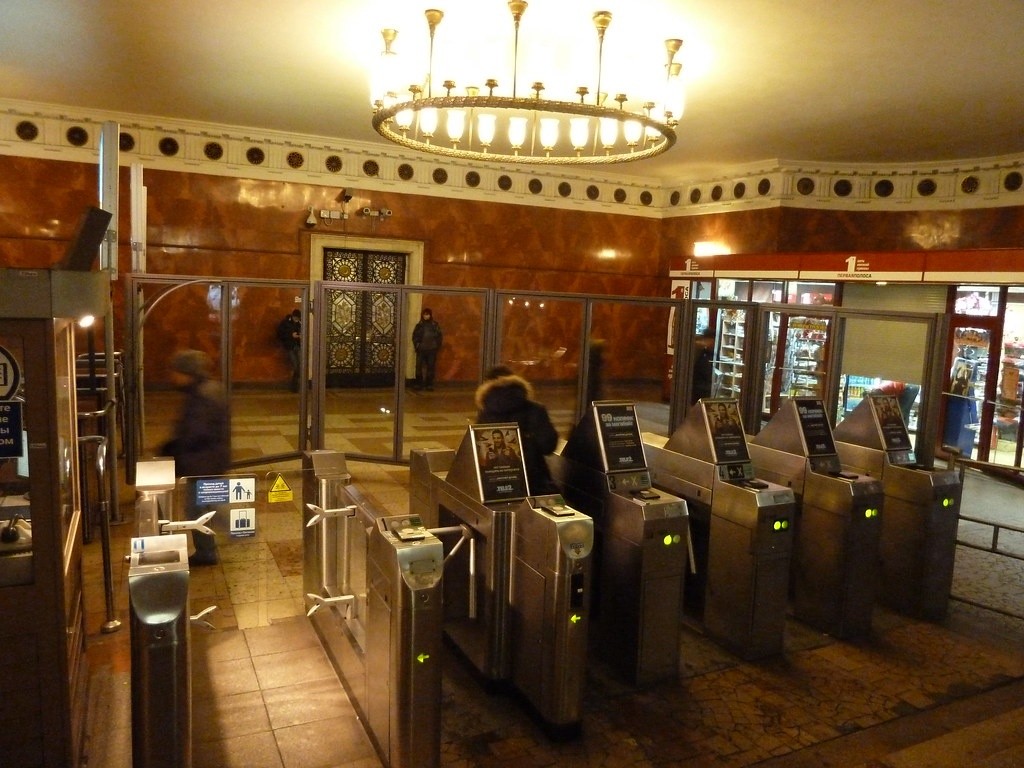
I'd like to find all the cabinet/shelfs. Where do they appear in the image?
[788,338,826,396]
[718,321,745,396]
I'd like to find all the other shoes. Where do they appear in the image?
[189,553,219,567]
[414,385,422,390]
[423,386,433,391]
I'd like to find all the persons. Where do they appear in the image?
[588,339,609,415]
[278,310,304,393]
[877,396,902,427]
[411,308,444,390]
[150,350,235,567]
[714,403,741,436]
[484,430,522,470]
[470,364,560,501]
[690,330,715,405]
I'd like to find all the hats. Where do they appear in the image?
[166,351,213,381]
[421,308,432,315]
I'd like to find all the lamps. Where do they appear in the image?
[369,0,686,166]
